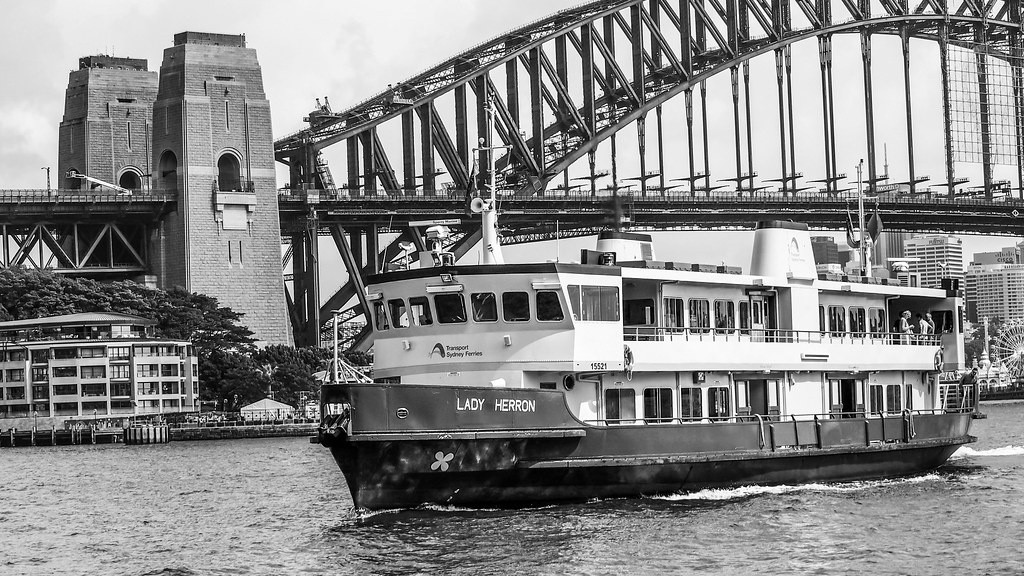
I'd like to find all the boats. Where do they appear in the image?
[311,205,984,520]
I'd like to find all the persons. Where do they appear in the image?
[899,310,935,345]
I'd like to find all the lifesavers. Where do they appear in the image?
[624,345,634,370]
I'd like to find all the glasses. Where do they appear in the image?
[972,370,976,374]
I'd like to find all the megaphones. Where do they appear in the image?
[471,198,488,212]
